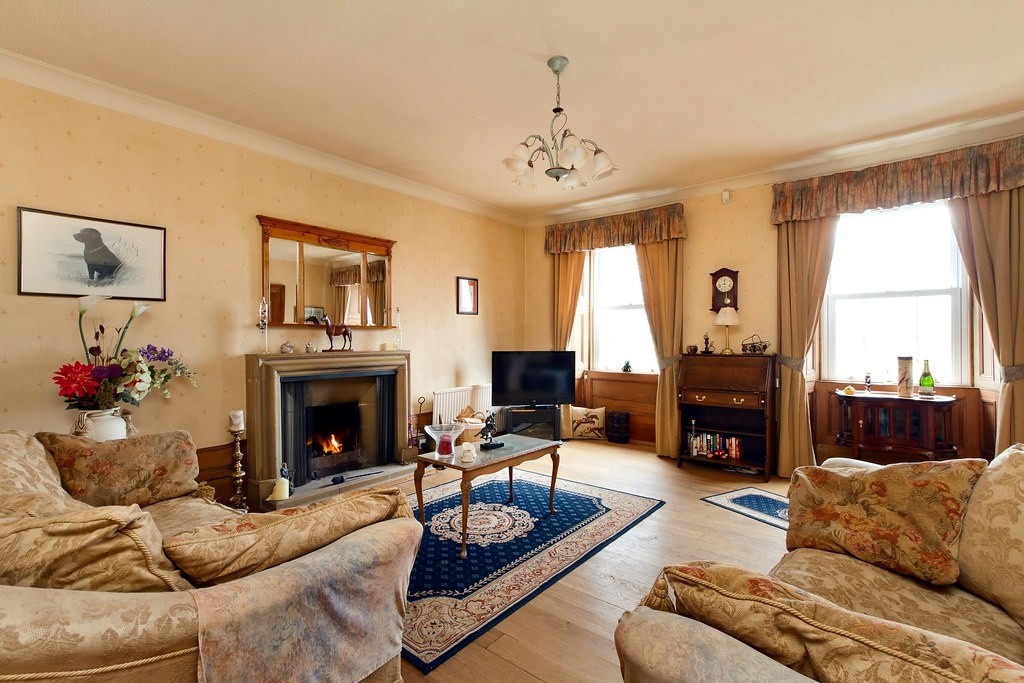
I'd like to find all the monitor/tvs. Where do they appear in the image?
[492,351,576,410]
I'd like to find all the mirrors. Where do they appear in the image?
[255,214,396,330]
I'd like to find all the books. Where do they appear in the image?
[687,433,745,462]
[843,404,945,443]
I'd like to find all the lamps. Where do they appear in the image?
[501,56,620,192]
[713,307,742,354]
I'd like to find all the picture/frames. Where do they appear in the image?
[16,204,167,300]
[457,276,479,316]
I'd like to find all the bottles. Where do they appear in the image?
[395,306,403,351]
[258,297,269,354]
[920,360,935,401]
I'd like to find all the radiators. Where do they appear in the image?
[430,384,508,442]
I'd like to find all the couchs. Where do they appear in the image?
[1,428,425,683]
[614,441,1024,683]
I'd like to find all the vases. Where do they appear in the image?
[68,407,139,443]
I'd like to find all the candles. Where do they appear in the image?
[228,409,244,432]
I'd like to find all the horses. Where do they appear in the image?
[305,315,319,324]
[320,313,353,350]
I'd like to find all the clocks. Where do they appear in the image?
[709,267,741,314]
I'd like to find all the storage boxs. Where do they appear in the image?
[452,412,487,447]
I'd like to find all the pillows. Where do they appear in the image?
[571,405,606,441]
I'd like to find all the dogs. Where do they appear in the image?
[72,228,123,279]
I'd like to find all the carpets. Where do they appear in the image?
[699,485,789,531]
[402,467,666,676]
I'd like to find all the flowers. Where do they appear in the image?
[53,288,200,410]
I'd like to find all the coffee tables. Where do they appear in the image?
[413,433,563,559]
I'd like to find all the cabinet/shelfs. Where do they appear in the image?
[675,386,777,484]
[835,388,963,463]
